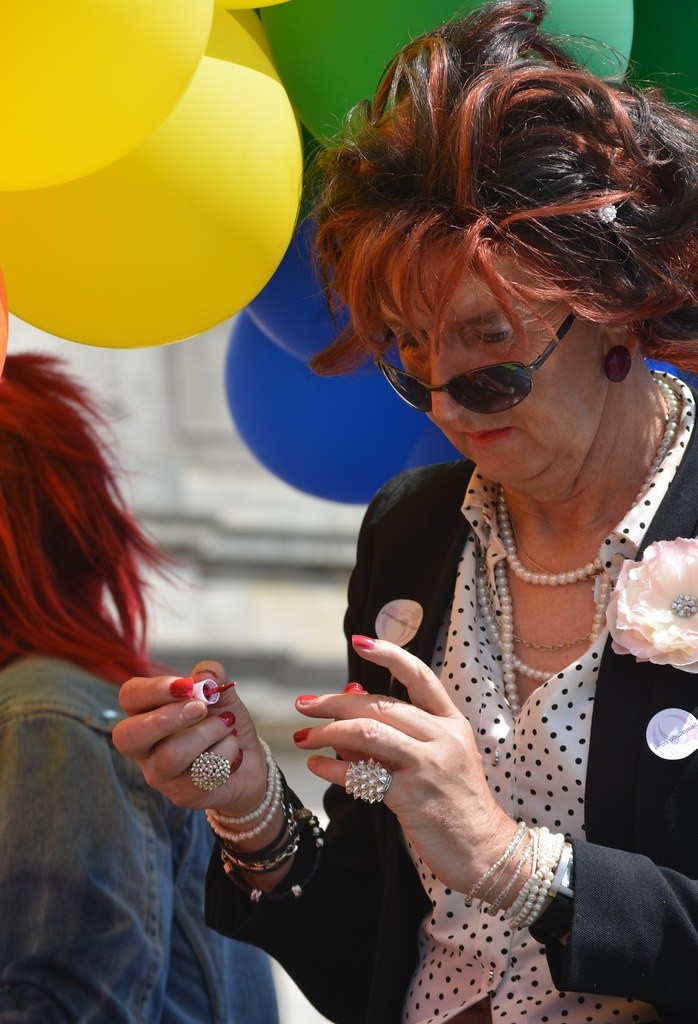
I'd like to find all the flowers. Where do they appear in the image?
[602,535,698,674]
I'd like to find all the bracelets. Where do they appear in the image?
[207,734,324,902]
[464,822,576,944]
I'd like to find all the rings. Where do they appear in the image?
[345,757,393,804]
[191,751,231,792]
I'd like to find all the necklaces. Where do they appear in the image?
[498,376,681,587]
[476,534,612,725]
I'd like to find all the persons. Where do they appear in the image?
[0,353,279,1024]
[111,0,698,1024]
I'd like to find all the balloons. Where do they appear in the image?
[0,0,698,504]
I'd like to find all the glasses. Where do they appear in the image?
[375,312,577,414]
